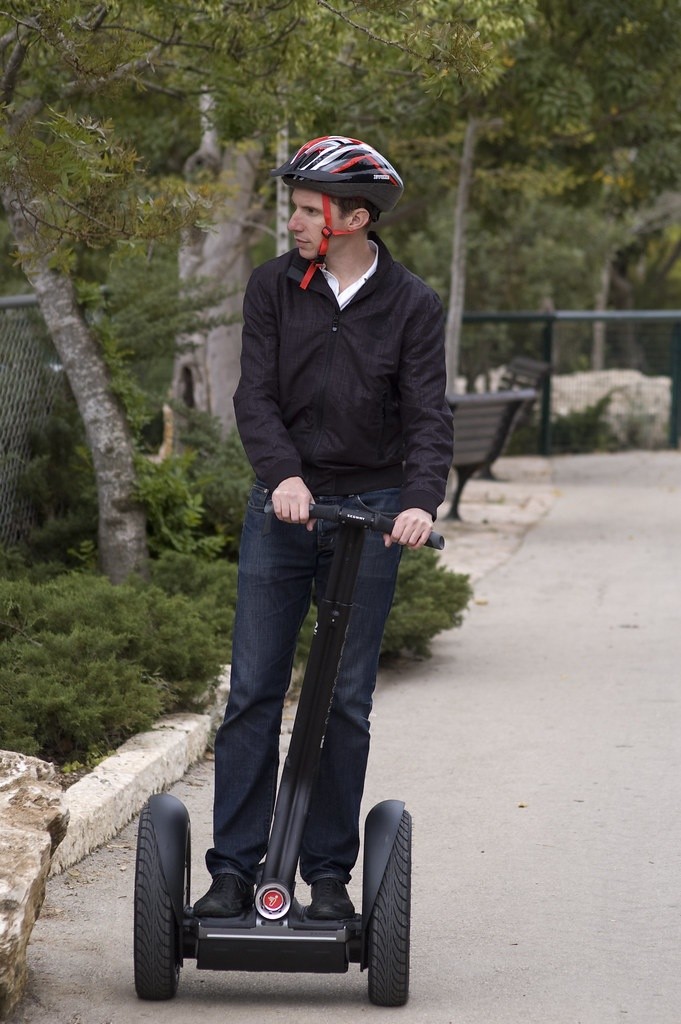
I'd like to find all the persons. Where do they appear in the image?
[191,132,453,922]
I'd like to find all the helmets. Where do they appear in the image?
[268,134,404,213]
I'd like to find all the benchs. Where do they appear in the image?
[441,355,546,524]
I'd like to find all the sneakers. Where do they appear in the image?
[193,872,255,919]
[306,878,355,921]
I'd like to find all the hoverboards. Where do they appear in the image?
[131,503,443,1009]
